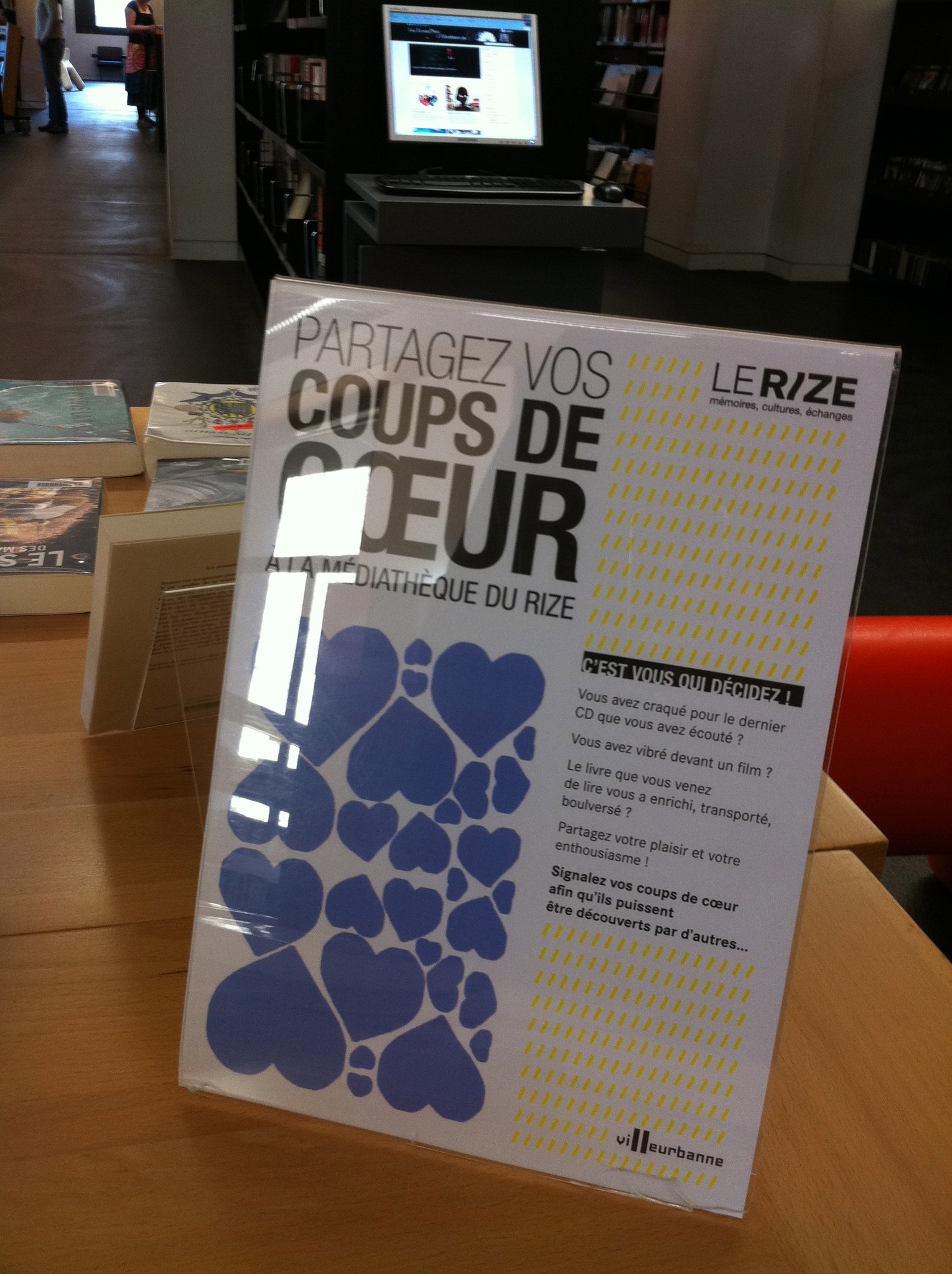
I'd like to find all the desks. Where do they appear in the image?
[0,853,952,1274]
[0,407,888,944]
[342,168,649,311]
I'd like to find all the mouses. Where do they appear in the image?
[593,181,625,203]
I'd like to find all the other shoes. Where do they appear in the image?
[38,120,56,131]
[49,124,69,134]
[137,116,157,129]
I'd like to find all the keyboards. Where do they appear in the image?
[376,174,584,199]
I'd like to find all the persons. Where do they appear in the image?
[35,0,68,134]
[125,0,163,129]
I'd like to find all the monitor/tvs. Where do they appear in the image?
[383,4,543,147]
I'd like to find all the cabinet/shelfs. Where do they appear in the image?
[234,0,384,299]
[585,0,673,209]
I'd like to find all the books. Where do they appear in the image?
[0,477,105,616]
[0,379,147,481]
[267,55,325,101]
[143,381,258,481]
[586,5,668,208]
[143,457,249,512]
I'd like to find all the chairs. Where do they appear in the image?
[92,46,128,83]
[824,615,952,878]
[61,48,86,90]
